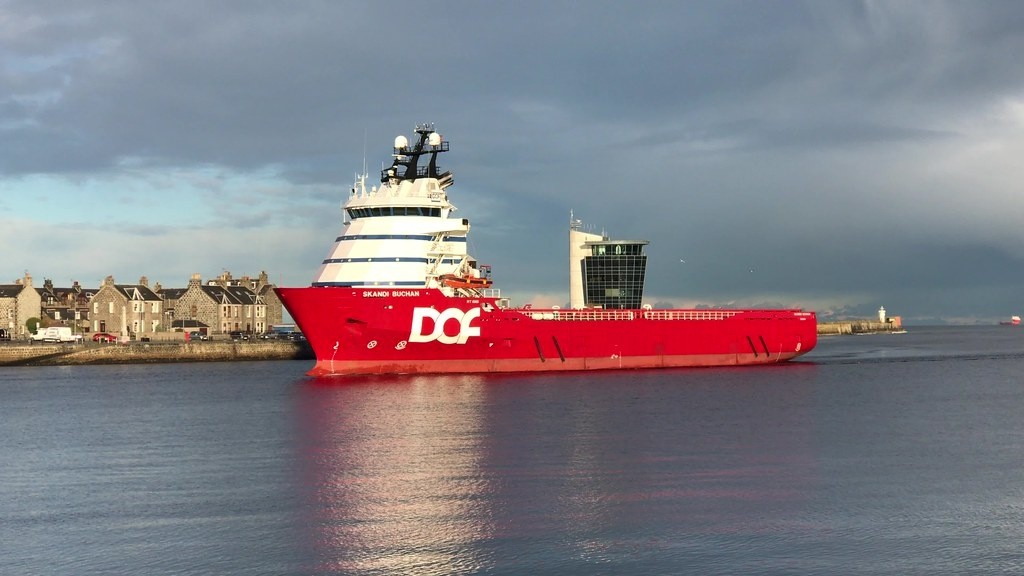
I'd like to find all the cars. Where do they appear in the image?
[229,330,251,342]
[188,331,208,341]
[93,333,117,342]
[0,328,11,342]
[258,328,308,344]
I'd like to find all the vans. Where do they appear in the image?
[29,326,84,345]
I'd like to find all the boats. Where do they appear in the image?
[271,120,819,381]
